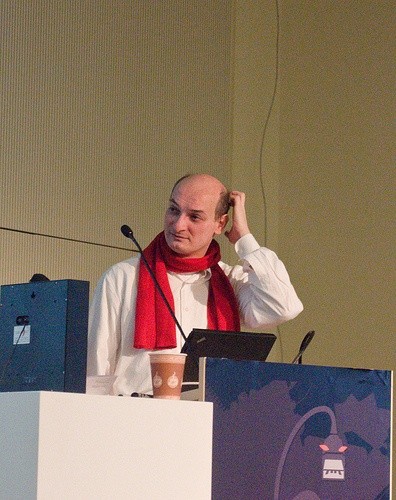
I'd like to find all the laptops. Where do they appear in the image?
[180,328,277,393]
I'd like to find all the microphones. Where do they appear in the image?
[121,224,200,369]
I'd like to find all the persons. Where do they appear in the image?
[87,174,304,398]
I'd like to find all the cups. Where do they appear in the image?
[149,354,187,400]
